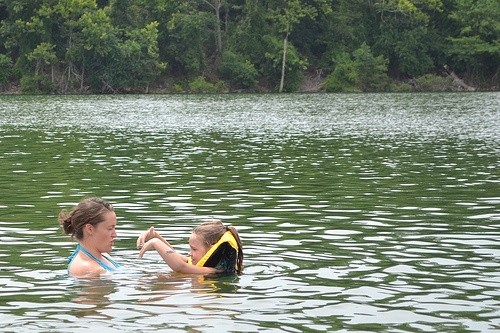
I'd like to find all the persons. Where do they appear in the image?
[57,196,119,276]
[136,221,243,278]
